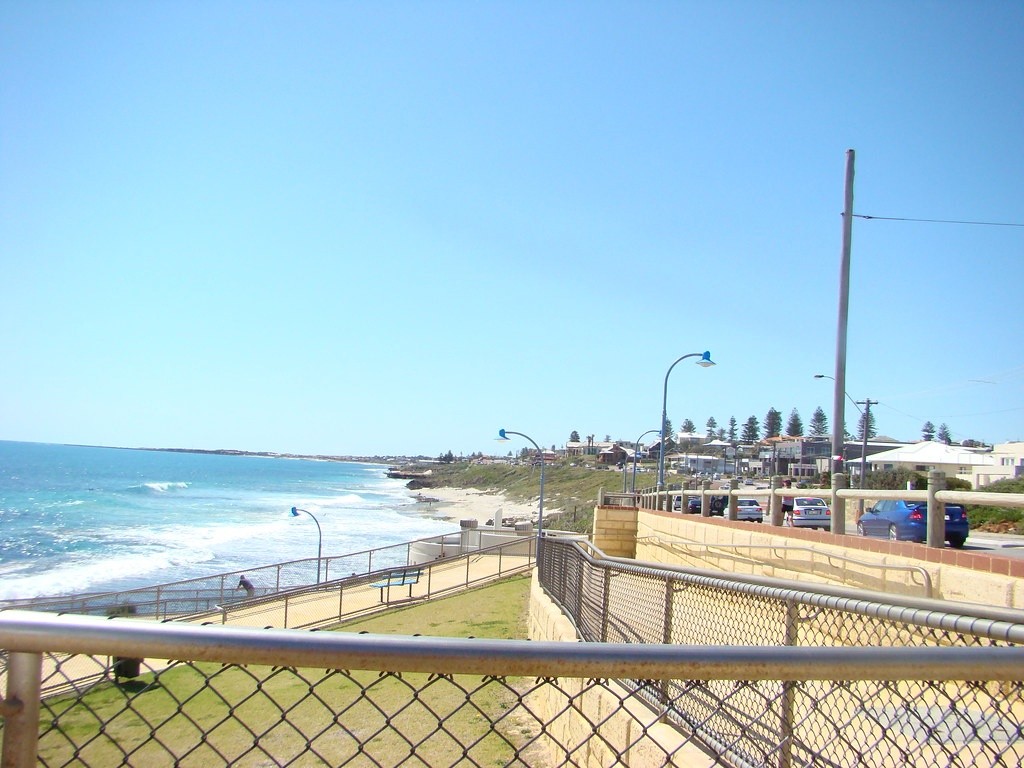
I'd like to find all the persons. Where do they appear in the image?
[236,575,255,597]
[782,480,794,527]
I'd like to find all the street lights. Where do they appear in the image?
[657,349,716,487]
[632,430,663,493]
[815,374,870,525]
[622,452,642,493]
[289,506,321,584]
[493,429,544,567]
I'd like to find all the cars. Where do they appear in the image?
[573,460,622,472]
[626,464,754,516]
[723,499,764,523]
[781,496,831,532]
[856,499,969,549]
